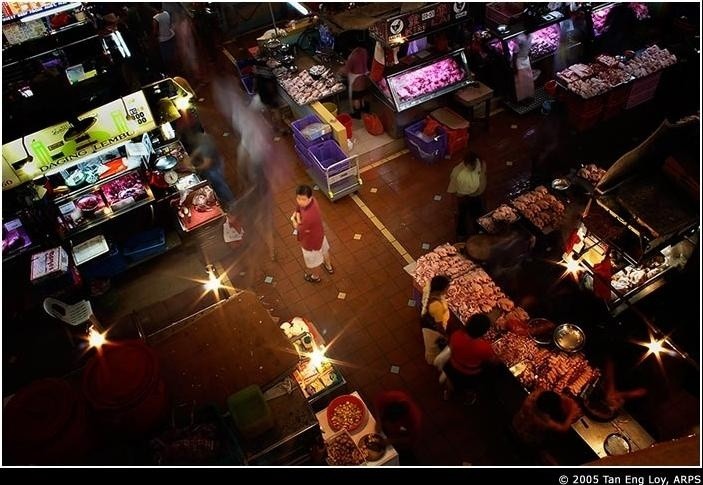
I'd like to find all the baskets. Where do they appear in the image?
[405,120,447,163]
[290,113,351,189]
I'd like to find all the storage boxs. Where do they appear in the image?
[29,245,69,286]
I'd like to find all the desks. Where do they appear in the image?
[453,80,494,135]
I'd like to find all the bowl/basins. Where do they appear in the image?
[527,317,586,353]
[327,394,366,433]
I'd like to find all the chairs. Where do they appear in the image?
[44,298,111,346]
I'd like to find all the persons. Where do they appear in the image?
[139,3,702,469]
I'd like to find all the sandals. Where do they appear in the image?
[304,274,321,283]
[324,265,335,275]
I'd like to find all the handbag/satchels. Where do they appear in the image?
[419,302,438,332]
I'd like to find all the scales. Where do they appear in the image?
[154,155,179,184]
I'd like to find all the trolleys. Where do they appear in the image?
[304,154,363,203]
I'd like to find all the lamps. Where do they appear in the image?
[286,2,313,16]
[17,3,82,25]
